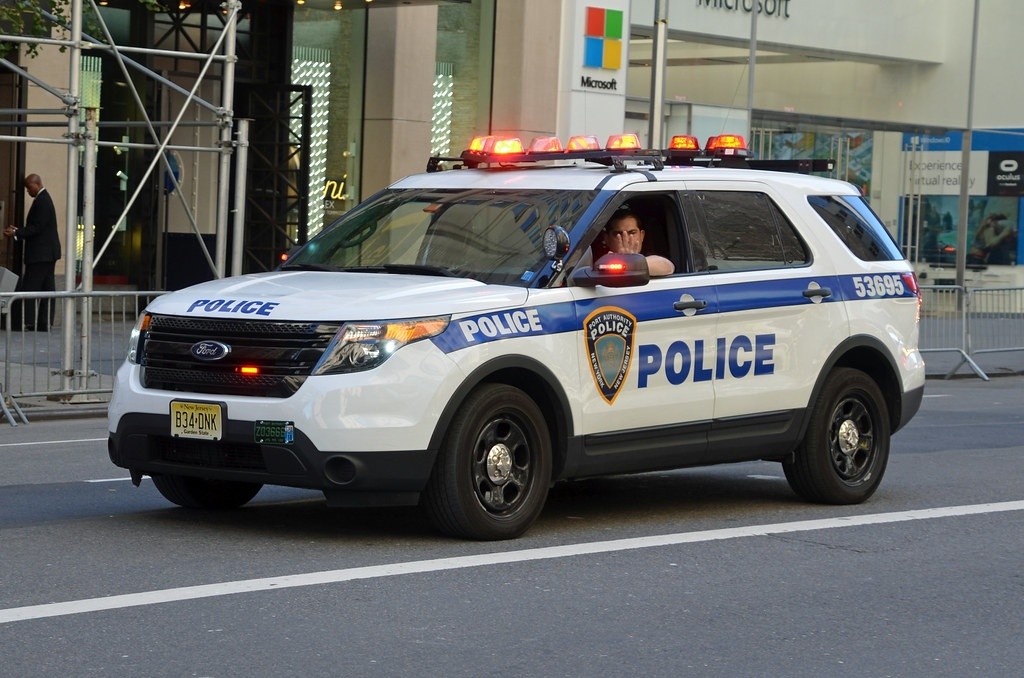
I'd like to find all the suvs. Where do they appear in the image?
[104,131,928,542]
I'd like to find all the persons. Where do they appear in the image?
[966,214,1013,265]
[604,208,675,276]
[3,174,61,332]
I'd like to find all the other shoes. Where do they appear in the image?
[1,323,22,331]
[28,323,51,332]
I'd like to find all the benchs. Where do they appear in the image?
[928,264,986,292]
